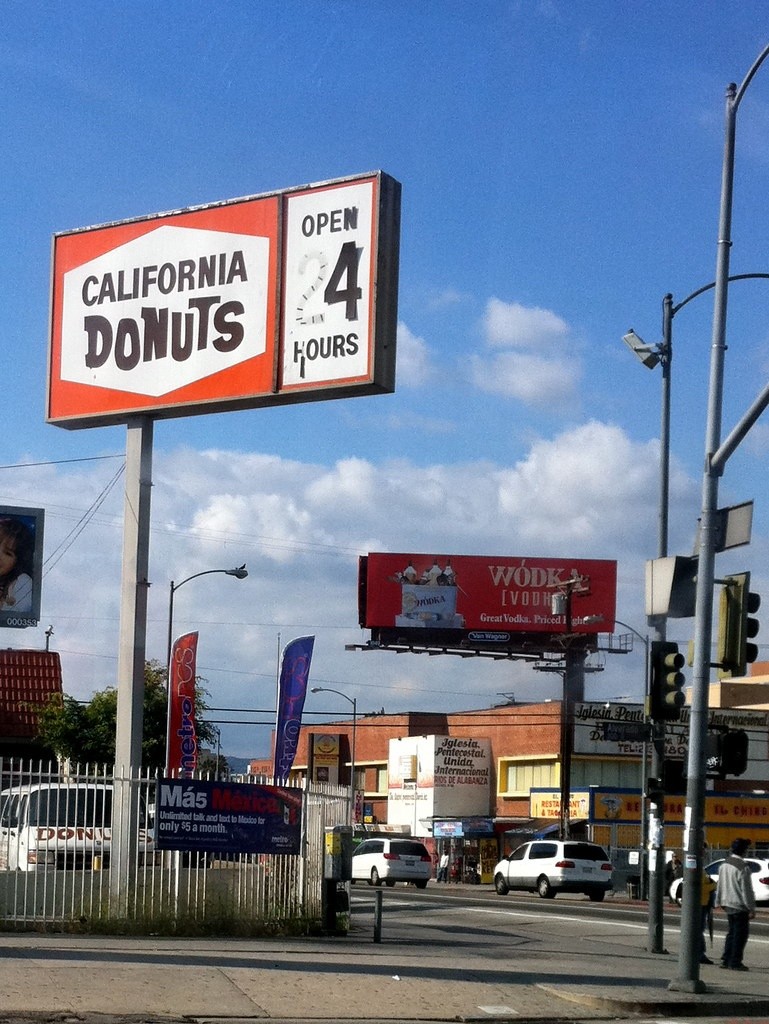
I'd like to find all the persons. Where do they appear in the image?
[701,840,717,964]
[715,838,756,971]
[665,853,683,903]
[435,847,449,883]
[0,513,34,612]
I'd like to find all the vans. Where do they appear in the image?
[0,783,164,875]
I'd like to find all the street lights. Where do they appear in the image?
[583,613,652,899]
[311,687,357,827]
[163,564,249,777]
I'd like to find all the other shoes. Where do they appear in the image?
[699,953,749,972]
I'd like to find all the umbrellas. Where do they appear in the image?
[707,910,713,949]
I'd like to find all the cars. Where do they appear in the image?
[668,858,769,908]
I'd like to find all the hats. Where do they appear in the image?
[731,837,752,849]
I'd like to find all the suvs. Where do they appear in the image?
[351,836,432,890]
[493,839,614,902]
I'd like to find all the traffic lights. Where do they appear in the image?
[650,641,686,720]
[710,730,750,773]
[721,571,762,677]
